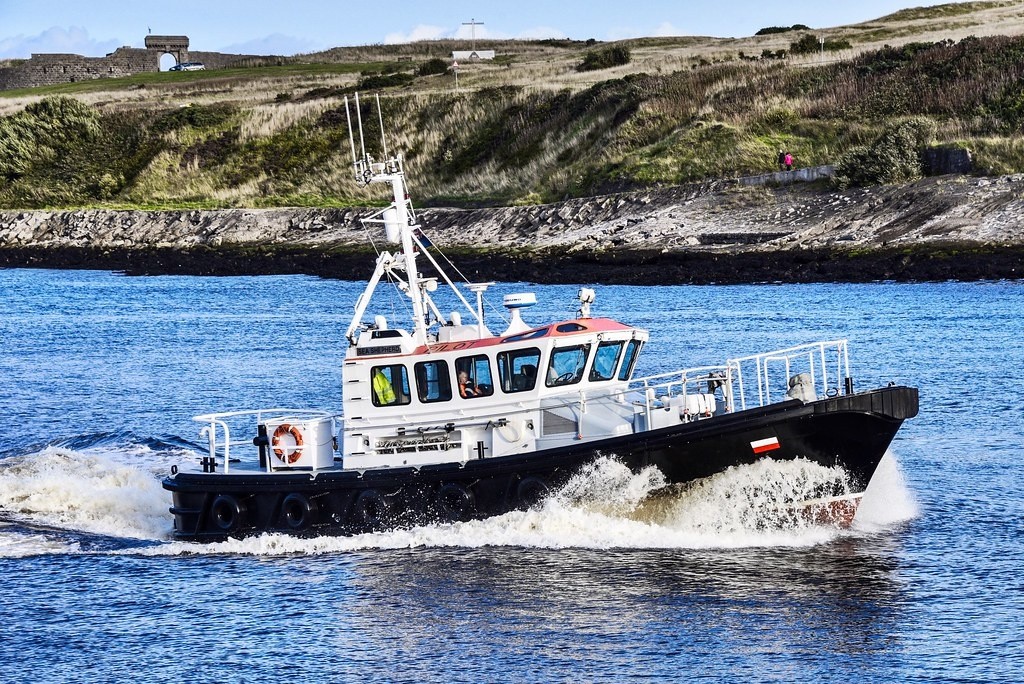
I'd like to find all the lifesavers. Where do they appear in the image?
[355,488,396,530]
[435,482,475,520]
[282,492,326,531]
[212,495,250,532]
[272,424,304,462]
[517,477,551,511]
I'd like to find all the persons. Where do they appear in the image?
[458,372,482,397]
[778,149,792,172]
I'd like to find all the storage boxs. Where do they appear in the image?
[265,417,334,469]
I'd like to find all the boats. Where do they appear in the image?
[153,87,923,549]
